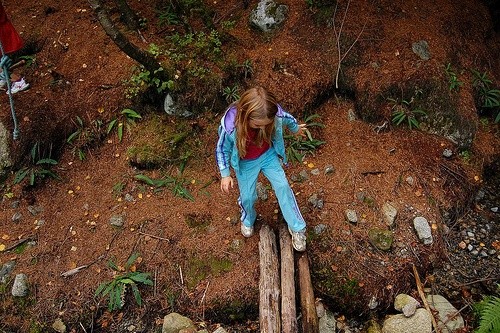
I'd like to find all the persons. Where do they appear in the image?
[216,86,307,252]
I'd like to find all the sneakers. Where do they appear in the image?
[6,77,30,95]
[287,224,307,251]
[240,220,254,237]
